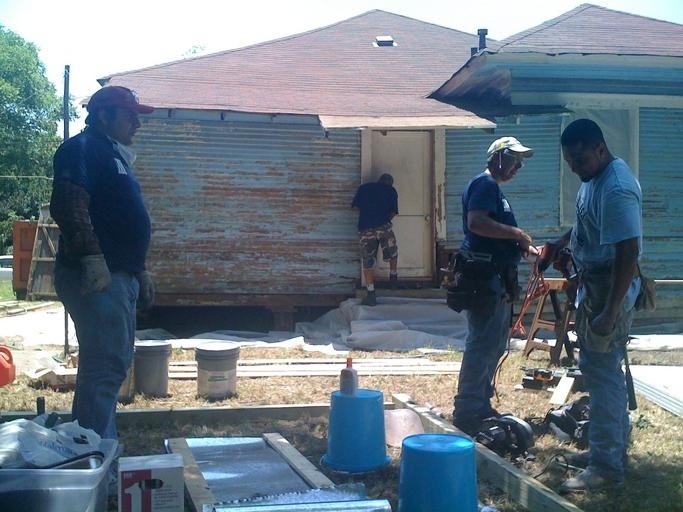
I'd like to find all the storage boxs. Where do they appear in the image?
[0,429,126,512]
[112,451,191,512]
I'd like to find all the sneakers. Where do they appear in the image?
[390,281,406,291]
[360,295,377,307]
[563,451,592,466]
[561,465,626,492]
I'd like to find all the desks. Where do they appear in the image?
[522,276,682,366]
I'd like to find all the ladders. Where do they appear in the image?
[522,277,578,362]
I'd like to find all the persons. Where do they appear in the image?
[536,117,643,494]
[50,86,155,440]
[350,173,405,305]
[451,135,540,439]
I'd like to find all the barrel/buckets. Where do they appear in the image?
[319,388,392,475]
[133,340,172,398]
[0,343,16,390]
[116,359,135,406]
[397,432,479,512]
[194,341,241,401]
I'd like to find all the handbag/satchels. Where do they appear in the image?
[576,296,628,352]
[634,276,658,312]
[440,251,503,319]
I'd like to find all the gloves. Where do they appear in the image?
[79,253,113,294]
[135,271,155,307]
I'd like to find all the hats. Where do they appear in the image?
[85,86,154,114]
[486,136,534,159]
